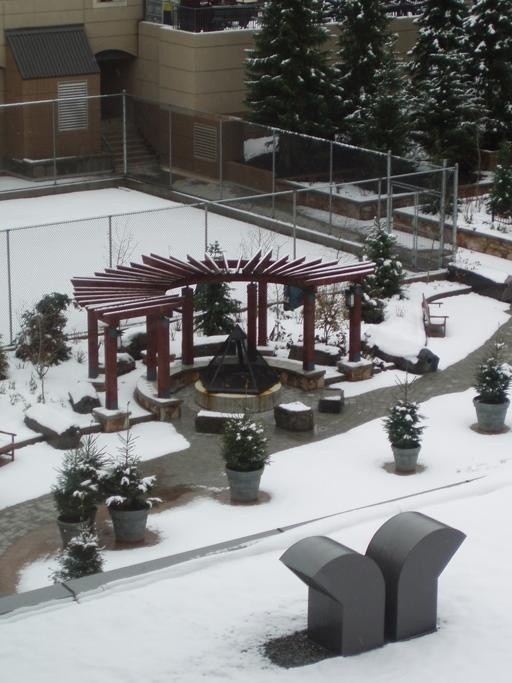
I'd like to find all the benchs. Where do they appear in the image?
[0,429,17,465]
[422,292,447,337]
[135,357,325,423]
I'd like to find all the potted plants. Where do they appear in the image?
[98,400,167,541]
[471,320,511,430]
[218,376,273,501]
[53,420,115,547]
[379,361,431,471]
